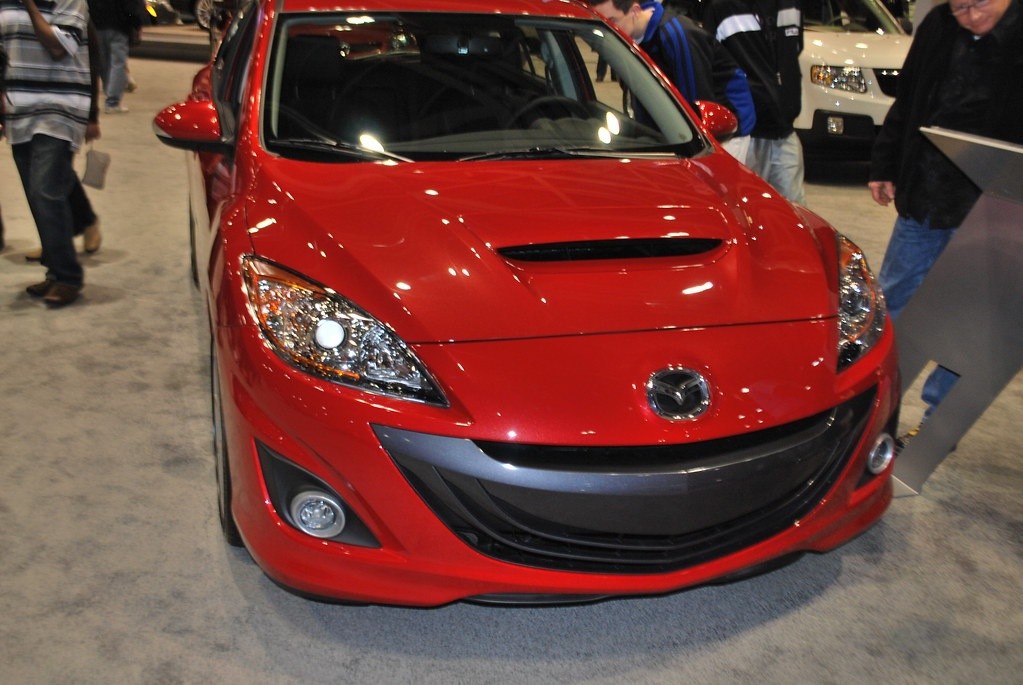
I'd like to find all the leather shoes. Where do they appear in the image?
[42,279,84,307]
[83,214,103,253]
[26,279,50,296]
[25,246,43,262]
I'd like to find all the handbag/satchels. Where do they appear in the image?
[81,138,112,190]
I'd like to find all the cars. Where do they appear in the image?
[772,0,914,171]
[153,0,902,609]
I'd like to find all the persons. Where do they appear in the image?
[591,0,806,212]
[867,2,1023,458]
[0,1,145,307]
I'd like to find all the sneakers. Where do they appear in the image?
[893,423,961,459]
[105,103,131,115]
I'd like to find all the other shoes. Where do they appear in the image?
[123,73,139,93]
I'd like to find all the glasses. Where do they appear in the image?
[606,14,626,25]
[952,0,991,17]
